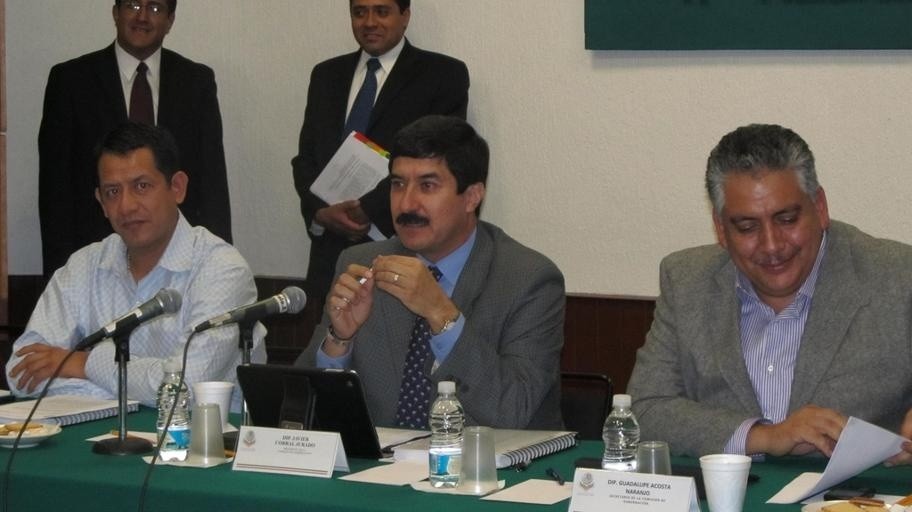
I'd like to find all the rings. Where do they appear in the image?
[393,274,399,283]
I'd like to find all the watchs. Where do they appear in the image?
[431,311,461,336]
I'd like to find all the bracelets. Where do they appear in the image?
[327,326,357,344]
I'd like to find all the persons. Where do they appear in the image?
[4,121,268,416]
[624,123,912,468]
[291,0,469,370]
[293,115,566,430]
[37,0,233,284]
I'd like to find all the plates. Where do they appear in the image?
[801,499,895,512]
[0,423,63,450]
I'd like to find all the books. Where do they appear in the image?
[380,425,580,469]
[0,395,141,427]
[309,130,396,241]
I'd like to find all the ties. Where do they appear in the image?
[345,58,381,131]
[129,62,155,127]
[394,263,444,430]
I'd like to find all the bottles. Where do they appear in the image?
[154,360,192,463]
[425,380,463,487]
[601,393,640,472]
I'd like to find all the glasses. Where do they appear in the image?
[125,1,169,13]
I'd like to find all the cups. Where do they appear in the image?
[188,401,227,465]
[698,454,752,512]
[637,440,672,477]
[456,425,502,493]
[193,381,234,428]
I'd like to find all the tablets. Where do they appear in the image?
[237,362,385,459]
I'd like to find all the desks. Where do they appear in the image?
[1,389,912,511]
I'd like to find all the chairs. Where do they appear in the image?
[556,372,616,440]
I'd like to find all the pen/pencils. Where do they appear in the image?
[336,255,382,312]
[224,449,237,457]
[111,430,156,444]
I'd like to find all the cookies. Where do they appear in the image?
[822,497,884,512]
[0,422,40,436]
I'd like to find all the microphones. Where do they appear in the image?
[75,288,182,351]
[195,287,307,332]
